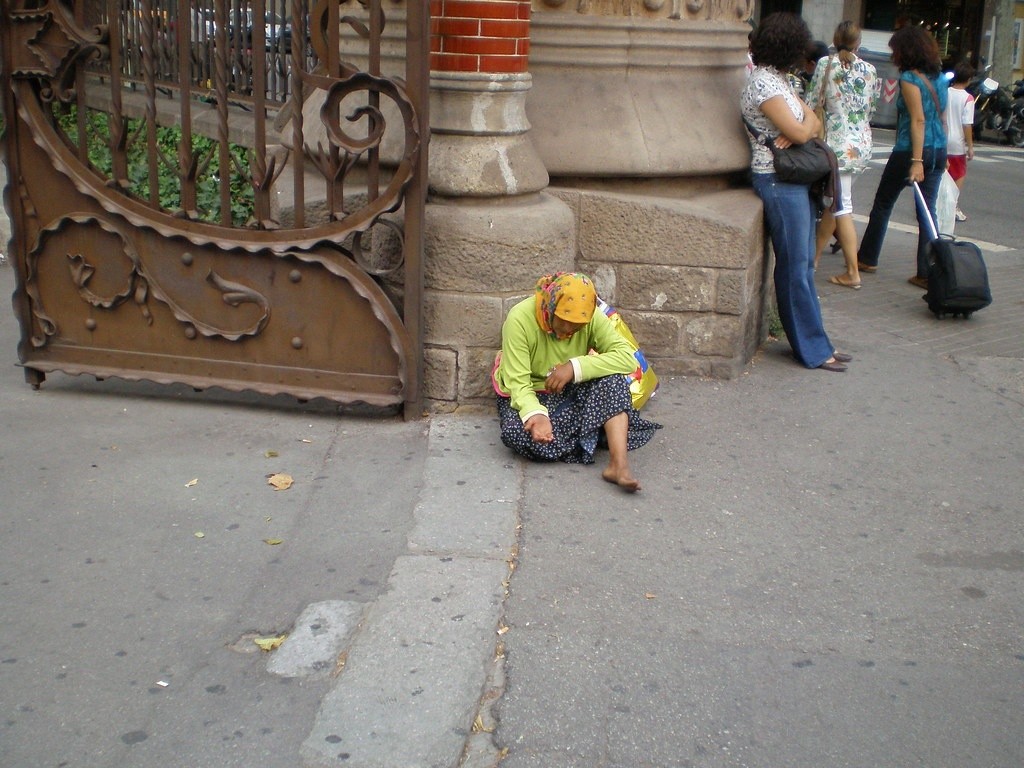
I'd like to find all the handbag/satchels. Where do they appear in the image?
[595,296,658,411]
[771,143,831,183]
[936,167,960,240]
[814,54,833,143]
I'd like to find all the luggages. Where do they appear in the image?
[906,175,992,320]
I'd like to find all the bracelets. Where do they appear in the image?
[911,158,924,162]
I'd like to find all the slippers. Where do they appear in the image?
[832,276,862,290]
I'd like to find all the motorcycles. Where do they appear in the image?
[944,55,1024,148]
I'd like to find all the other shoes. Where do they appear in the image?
[910,276,930,289]
[955,208,967,222]
[833,349,853,362]
[857,260,877,273]
[821,357,848,372]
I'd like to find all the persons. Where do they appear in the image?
[844,28,948,290]
[491,272,643,493]
[942,62,974,222]
[740,12,877,372]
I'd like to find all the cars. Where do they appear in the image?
[120,6,315,59]
